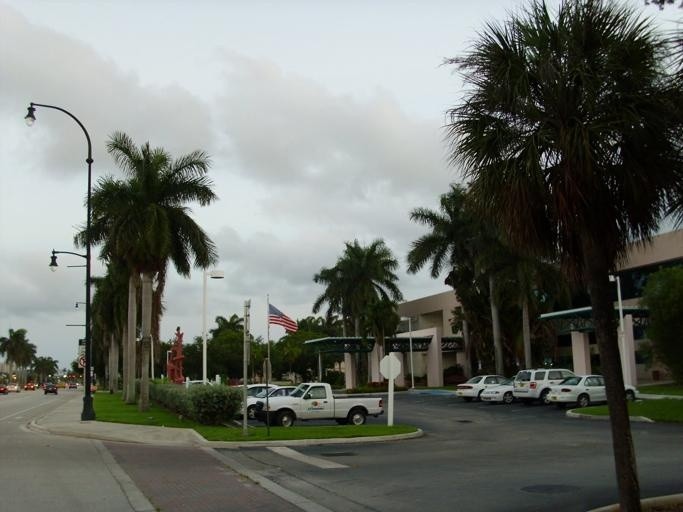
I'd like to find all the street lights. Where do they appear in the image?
[400,316,415,388]
[24,102,96,419]
[203,269,225,384]
[608,273,639,400]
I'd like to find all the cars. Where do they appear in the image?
[229,381,313,420]
[455,368,640,408]
[0,382,21,395]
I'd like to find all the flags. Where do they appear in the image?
[269,304,298,332]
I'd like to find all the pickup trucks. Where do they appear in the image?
[255,383,385,428]
